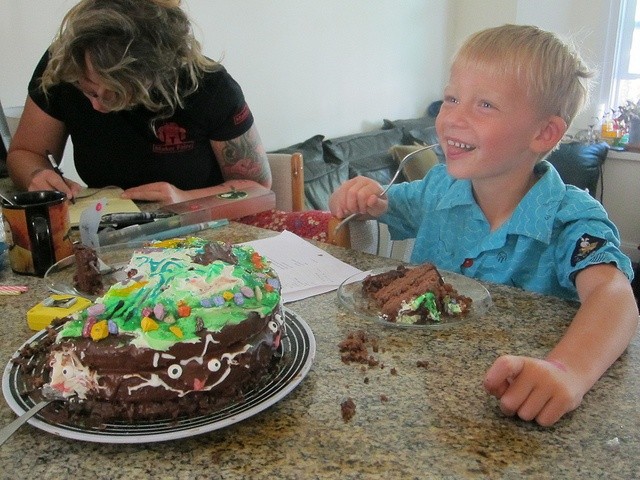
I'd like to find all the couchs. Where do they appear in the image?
[268,118,606,214]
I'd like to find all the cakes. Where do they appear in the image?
[35,236,287,419]
[361,263,473,325]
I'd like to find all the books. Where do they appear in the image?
[69,185,142,225]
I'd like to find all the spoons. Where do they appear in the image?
[331,143,442,234]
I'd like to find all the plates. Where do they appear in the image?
[336,267,492,331]
[42,244,151,306]
[0,305,319,444]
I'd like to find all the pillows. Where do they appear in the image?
[547,142,609,201]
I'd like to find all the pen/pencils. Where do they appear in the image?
[128,218,230,247]
[45,149,76,206]
[76,209,179,243]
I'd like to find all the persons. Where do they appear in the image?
[5,0,273,220]
[327,23,639,428]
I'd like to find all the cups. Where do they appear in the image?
[1,190,75,277]
